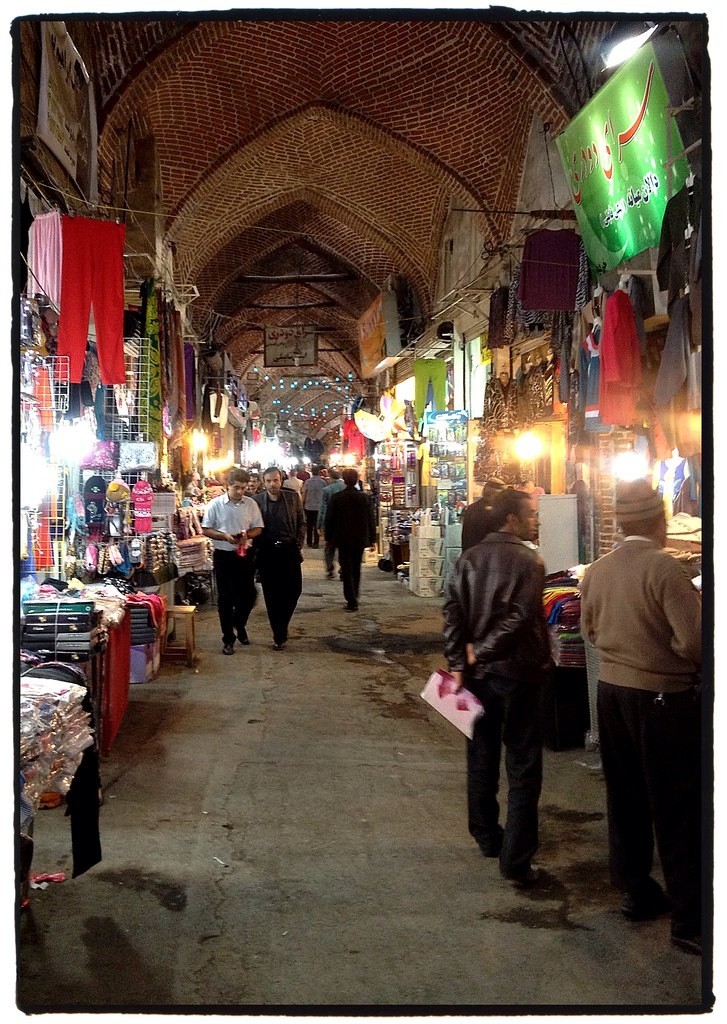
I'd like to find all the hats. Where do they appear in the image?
[665,512,702,541]
[79,441,119,471]
[131,480,155,502]
[104,478,133,533]
[83,475,106,500]
[614,480,666,526]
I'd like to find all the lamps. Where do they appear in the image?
[293,252,302,367]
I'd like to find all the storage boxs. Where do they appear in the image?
[409,525,462,597]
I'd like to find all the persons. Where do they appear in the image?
[461,478,506,554]
[250,466,307,650]
[522,480,546,511]
[202,469,264,655]
[443,490,556,887]
[324,468,377,610]
[580,479,702,954]
[570,480,600,565]
[216,463,363,580]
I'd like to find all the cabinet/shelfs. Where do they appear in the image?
[539,494,577,581]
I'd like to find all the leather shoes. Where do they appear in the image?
[273,642,286,651]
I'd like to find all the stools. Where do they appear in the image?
[164,604,199,667]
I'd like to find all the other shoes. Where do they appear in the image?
[502,862,536,885]
[620,886,672,923]
[236,629,250,645]
[671,925,702,956]
[221,644,236,656]
[326,572,334,578]
[342,605,359,612]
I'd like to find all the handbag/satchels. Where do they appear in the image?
[419,668,486,741]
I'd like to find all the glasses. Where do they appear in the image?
[517,510,540,519]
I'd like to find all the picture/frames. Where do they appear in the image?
[264,325,320,369]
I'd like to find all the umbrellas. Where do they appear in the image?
[381,396,409,434]
[354,410,389,441]
